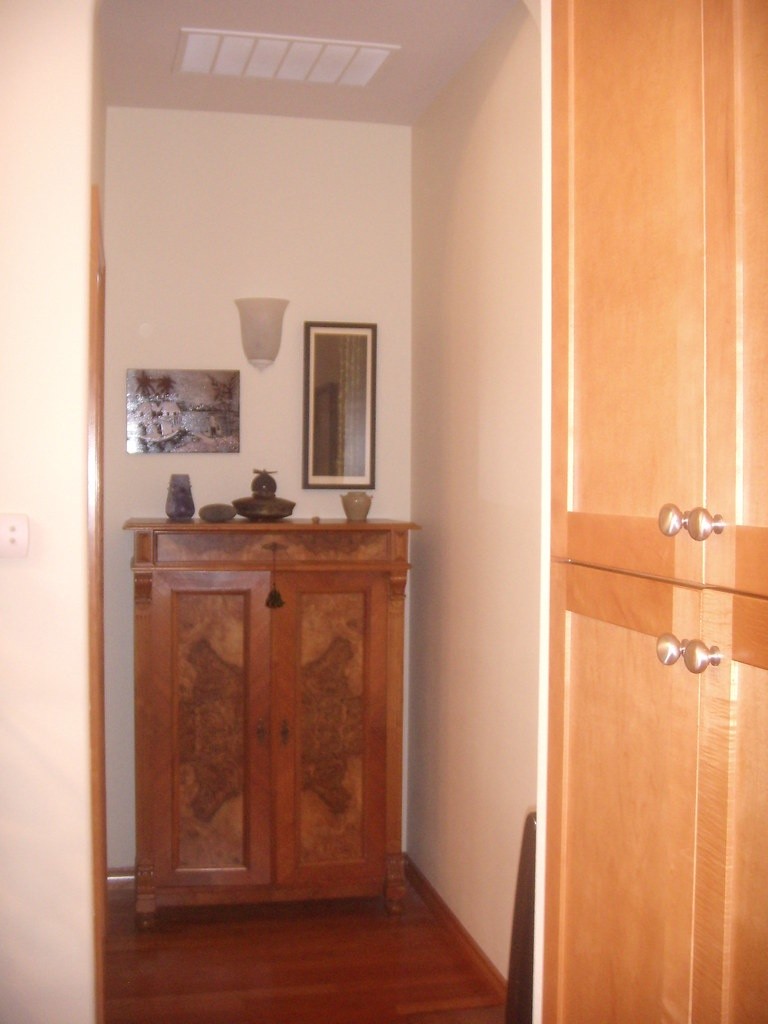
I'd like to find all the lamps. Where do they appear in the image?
[234,297,289,369]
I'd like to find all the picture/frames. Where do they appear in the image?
[302,321,377,490]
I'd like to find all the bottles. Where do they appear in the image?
[342,492,371,521]
[165,473,194,521]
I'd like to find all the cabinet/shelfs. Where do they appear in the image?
[544,0,768,1024]
[123,522,410,918]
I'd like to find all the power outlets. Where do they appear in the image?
[1,512,29,557]
